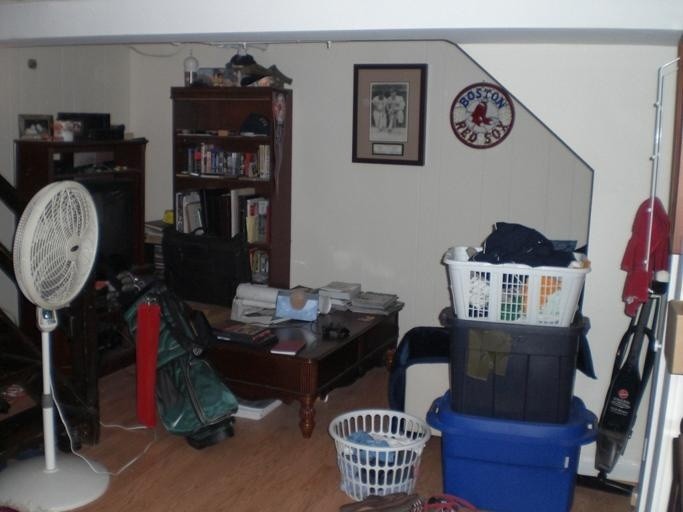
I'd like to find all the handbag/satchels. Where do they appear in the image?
[160,226,253,310]
[122,279,241,451]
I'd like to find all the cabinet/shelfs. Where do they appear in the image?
[13,137,146,379]
[169,85,293,309]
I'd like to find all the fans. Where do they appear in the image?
[0,180,111,512]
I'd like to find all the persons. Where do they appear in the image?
[386,88,405,128]
[372,89,390,129]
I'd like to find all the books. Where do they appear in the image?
[212,322,306,358]
[248,248,269,275]
[316,280,405,317]
[176,143,273,242]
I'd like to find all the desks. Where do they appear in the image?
[210,309,400,439]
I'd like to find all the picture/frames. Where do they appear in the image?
[351,63,428,167]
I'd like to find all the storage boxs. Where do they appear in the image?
[426,389,600,512]
[439,307,584,425]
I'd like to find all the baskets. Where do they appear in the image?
[442,245,591,328]
[328,408,432,503]
